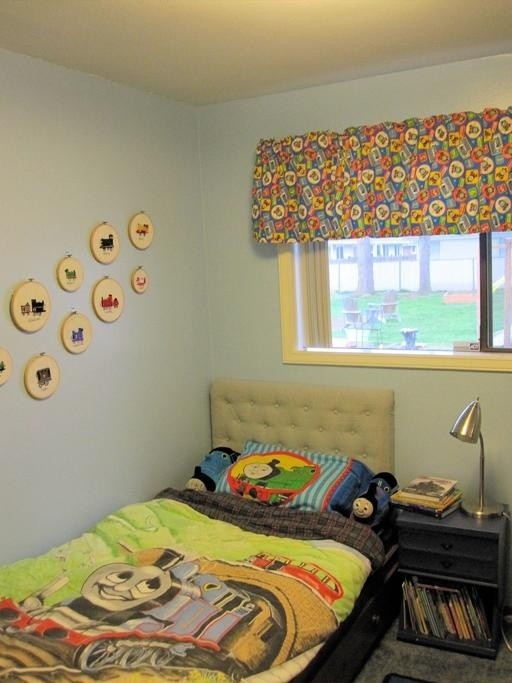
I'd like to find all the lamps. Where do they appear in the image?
[449,397,505,520]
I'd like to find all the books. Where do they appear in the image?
[389,473,464,519]
[402,574,492,641]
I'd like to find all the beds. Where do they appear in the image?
[0,377,399,683]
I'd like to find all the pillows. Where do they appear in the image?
[213,439,375,516]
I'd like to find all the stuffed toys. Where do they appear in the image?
[184,446,241,495]
[349,471,399,525]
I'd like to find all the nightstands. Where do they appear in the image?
[394,503,507,660]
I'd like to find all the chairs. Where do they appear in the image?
[341,289,401,345]
